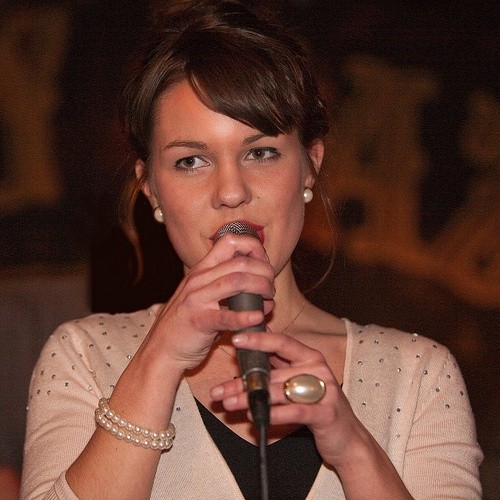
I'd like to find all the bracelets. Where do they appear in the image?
[97,397,176,441]
[95,408,173,450]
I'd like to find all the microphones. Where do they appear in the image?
[215,221,269,423]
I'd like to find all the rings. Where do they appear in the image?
[284,373,327,405]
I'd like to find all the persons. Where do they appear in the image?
[16,1,485,500]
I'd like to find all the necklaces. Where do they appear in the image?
[216,301,310,358]
